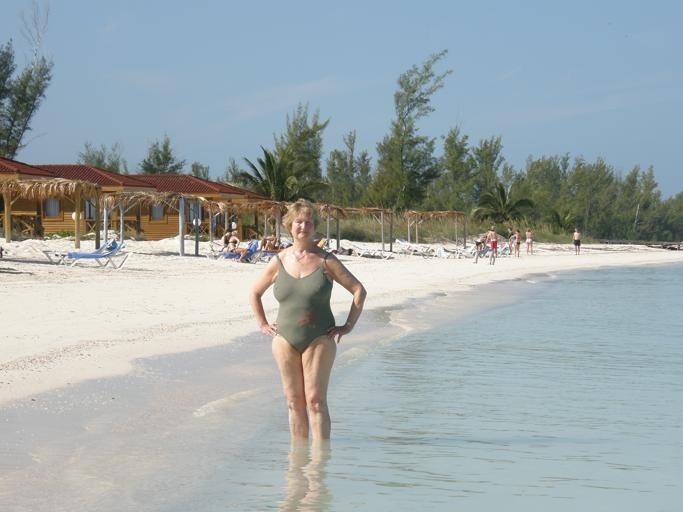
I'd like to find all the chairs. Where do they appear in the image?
[42,238,129,269]
[346,241,383,260]
[221,239,258,263]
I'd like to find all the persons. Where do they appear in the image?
[472,225,520,266]
[524,228,534,255]
[216,227,359,264]
[572,227,581,255]
[249,196,367,442]
